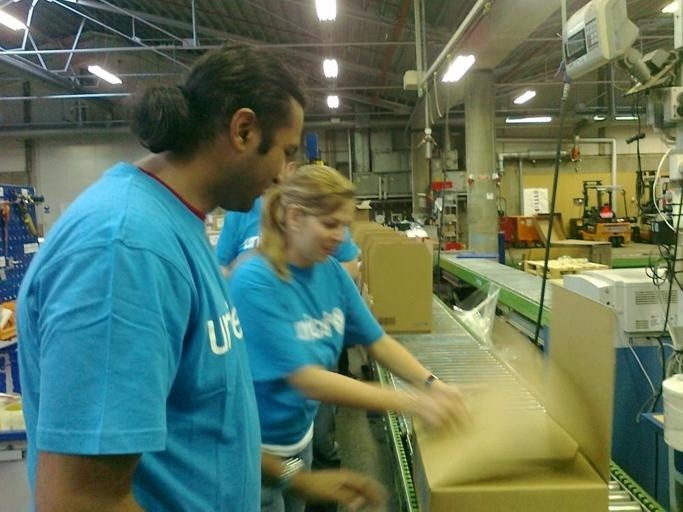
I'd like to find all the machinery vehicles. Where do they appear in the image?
[630,170,674,245]
[567,180,632,247]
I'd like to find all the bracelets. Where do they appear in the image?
[421,373,439,393]
[275,455,308,496]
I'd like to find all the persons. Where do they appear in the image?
[215,144,361,281]
[224,166,470,511]
[16,40,394,511]
